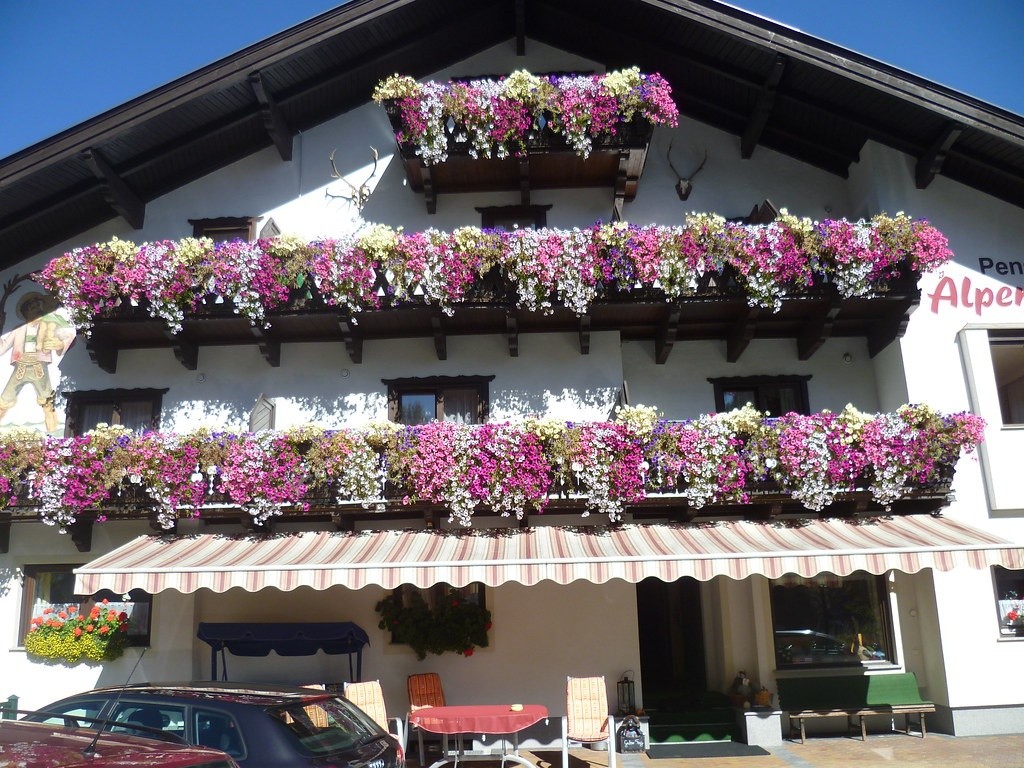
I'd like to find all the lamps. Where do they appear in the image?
[616,670,637,716]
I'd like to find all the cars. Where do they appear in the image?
[775,628,886,669]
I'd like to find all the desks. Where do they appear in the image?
[410,704,549,768]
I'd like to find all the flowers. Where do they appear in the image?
[0,404,989,536]
[374,590,492,661]
[372,66,680,168]
[24,599,136,663]
[29,207,954,339]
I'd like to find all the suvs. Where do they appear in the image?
[0,681,406,768]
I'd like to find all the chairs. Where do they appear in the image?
[127,709,162,738]
[561,675,616,768]
[344,679,405,754]
[406,673,448,765]
[286,684,328,727]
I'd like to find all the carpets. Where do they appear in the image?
[646,741,771,759]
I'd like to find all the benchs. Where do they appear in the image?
[776,672,937,746]
